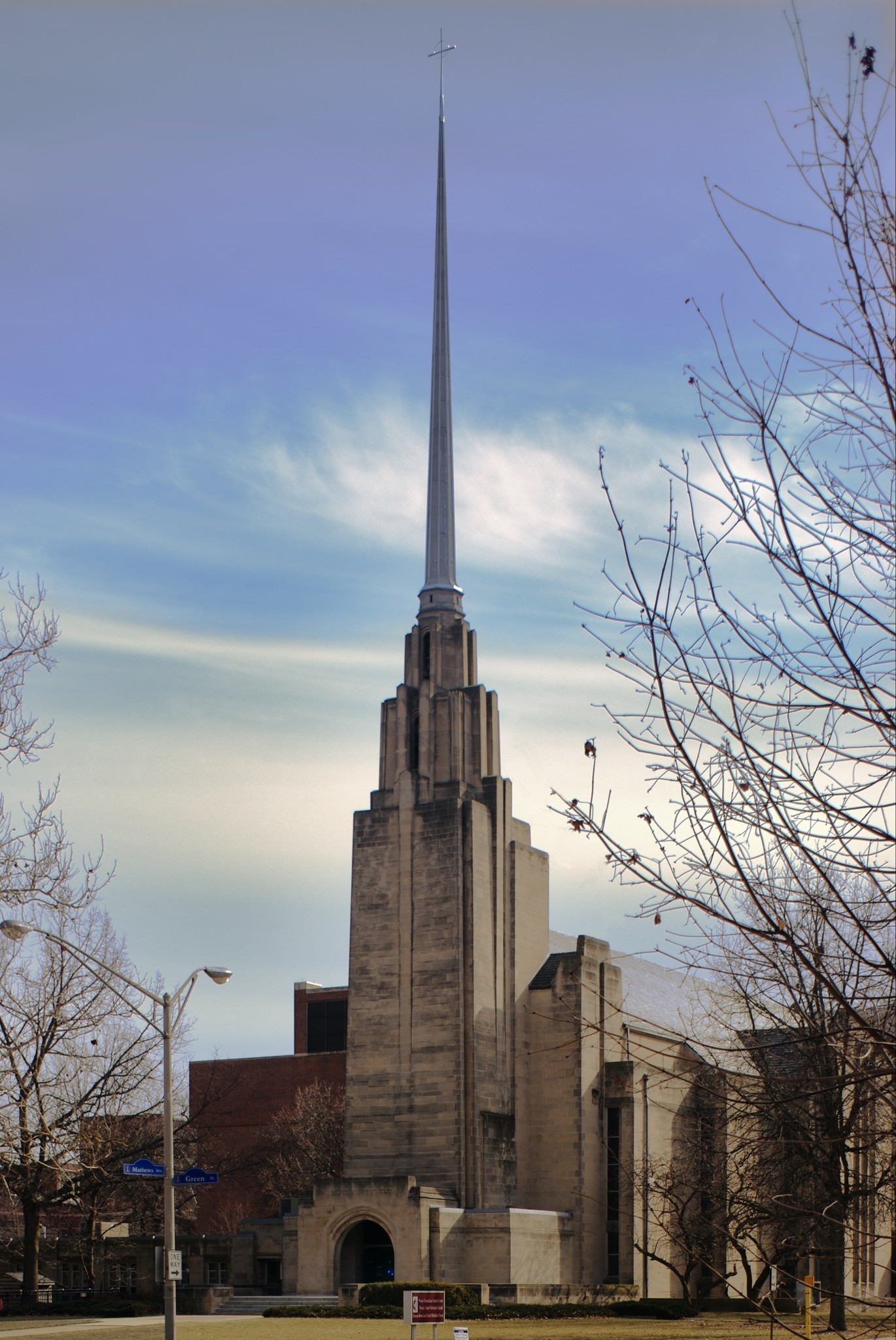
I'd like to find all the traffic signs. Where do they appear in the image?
[168,1251,182,1281]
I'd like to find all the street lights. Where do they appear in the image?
[1,921,232,1340]
